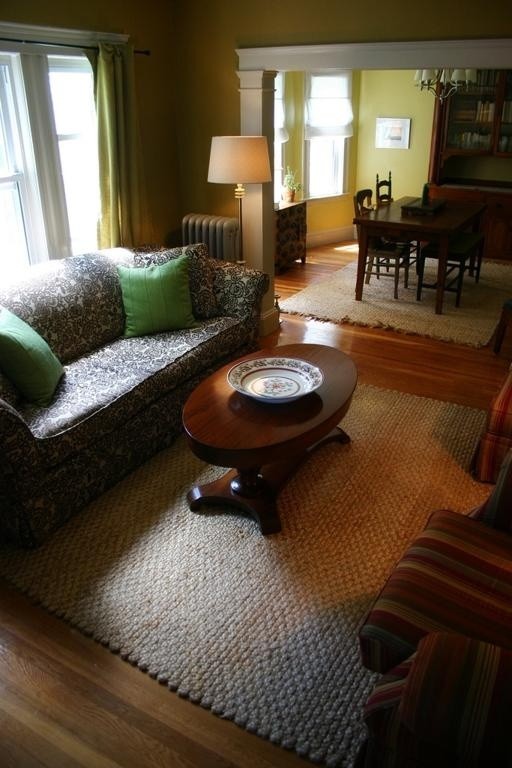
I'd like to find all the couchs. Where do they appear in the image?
[0,247,269,544]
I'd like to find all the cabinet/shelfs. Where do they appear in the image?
[427,69,512,190]
[275,201,307,276]
[419,184,512,261]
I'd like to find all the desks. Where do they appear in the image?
[353,196,487,315]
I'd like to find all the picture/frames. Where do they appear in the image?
[375,117,411,150]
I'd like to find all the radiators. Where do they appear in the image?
[180,213,242,264]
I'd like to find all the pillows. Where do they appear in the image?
[0,304,65,411]
[123,243,222,319]
[114,255,199,339]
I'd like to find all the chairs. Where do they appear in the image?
[375,172,419,279]
[416,203,484,307]
[354,510,512,768]
[471,360,512,484]
[353,189,410,299]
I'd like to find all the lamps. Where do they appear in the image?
[207,135,272,267]
[413,67,478,107]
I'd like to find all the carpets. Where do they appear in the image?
[1,384,494,768]
[275,254,512,348]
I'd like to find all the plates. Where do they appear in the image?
[227,357,325,403]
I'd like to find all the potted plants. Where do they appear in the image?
[282,166,302,203]
